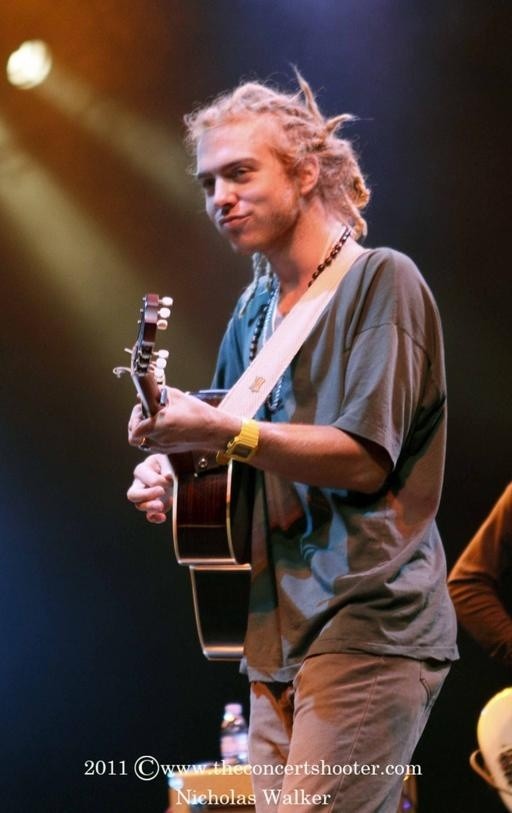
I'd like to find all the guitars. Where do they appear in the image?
[130,293,258,663]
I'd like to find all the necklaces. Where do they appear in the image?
[246,220,353,414]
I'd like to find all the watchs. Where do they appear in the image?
[221,416,260,462]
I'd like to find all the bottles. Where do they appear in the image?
[220,701,247,764]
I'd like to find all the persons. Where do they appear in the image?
[123,59,465,813]
[439,473,512,673]
